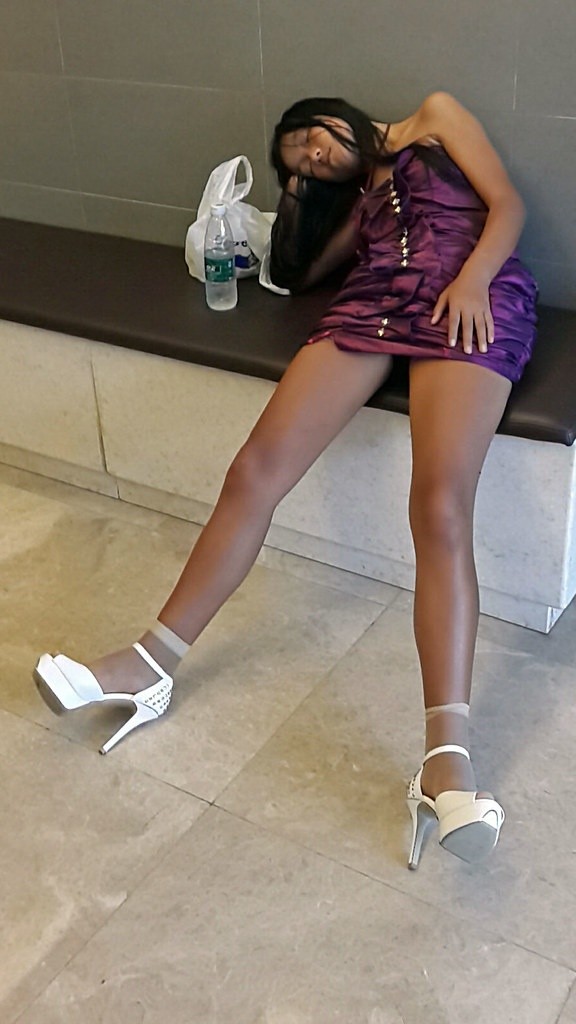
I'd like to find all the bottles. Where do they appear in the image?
[204,203,239,311]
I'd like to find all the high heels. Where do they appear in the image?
[396,742,507,876]
[29,641,174,759]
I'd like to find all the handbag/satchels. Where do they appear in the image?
[183,153,280,281]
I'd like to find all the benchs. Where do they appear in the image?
[0,215,574,636]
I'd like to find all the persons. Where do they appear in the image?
[32,84,537,874]
